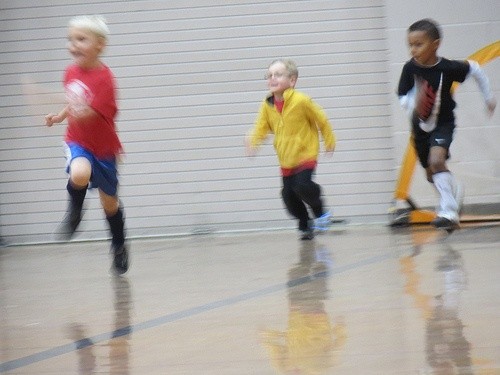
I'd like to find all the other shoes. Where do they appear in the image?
[110,245,129,275]
[301,218,315,242]
[56,210,82,242]
[307,181,323,218]
[432,215,460,229]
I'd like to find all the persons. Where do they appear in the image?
[396,18,497,232]
[244,58,337,240]
[46,14,130,275]
